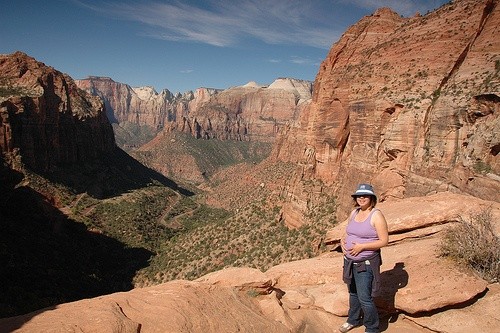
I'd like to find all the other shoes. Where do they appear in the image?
[339,321,355,333]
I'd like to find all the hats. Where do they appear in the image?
[350,183,377,201]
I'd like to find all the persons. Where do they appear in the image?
[340,183,390,333]
